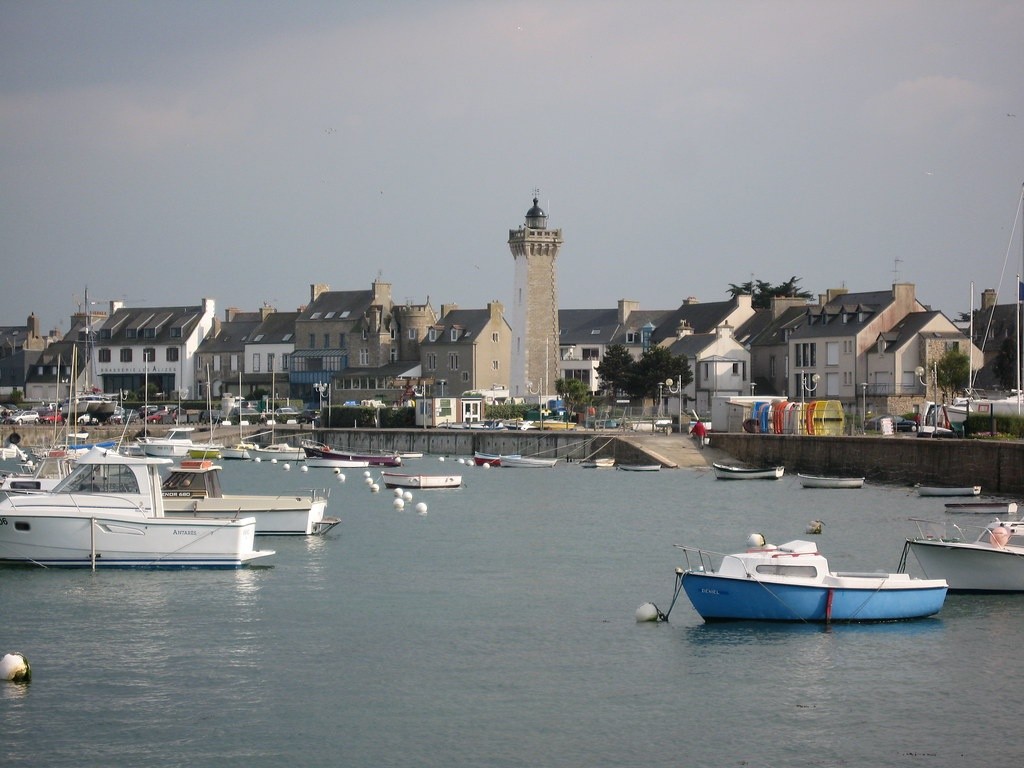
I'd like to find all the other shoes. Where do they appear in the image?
[702,446,703,449]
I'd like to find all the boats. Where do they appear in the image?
[472,450,522,466]
[577,457,615,468]
[673,532,949,622]
[712,462,786,480]
[904,517,1024,594]
[438,418,577,429]
[617,464,663,472]
[944,501,1018,514]
[498,453,558,468]
[1,342,463,571]
[914,483,981,496]
[796,472,867,490]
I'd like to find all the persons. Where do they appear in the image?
[172,409,177,424]
[689,420,707,448]
[1,409,7,418]
[198,411,204,422]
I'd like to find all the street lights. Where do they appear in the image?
[914,361,938,437]
[860,383,868,434]
[440,379,445,397]
[119,387,128,425]
[658,382,665,415]
[525,378,543,430]
[313,381,331,429]
[801,369,819,435]
[412,380,426,429]
[750,382,757,395]
[177,386,190,426]
[665,374,682,435]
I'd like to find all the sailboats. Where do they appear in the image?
[941,275,1024,433]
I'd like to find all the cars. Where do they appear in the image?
[870,415,918,432]
